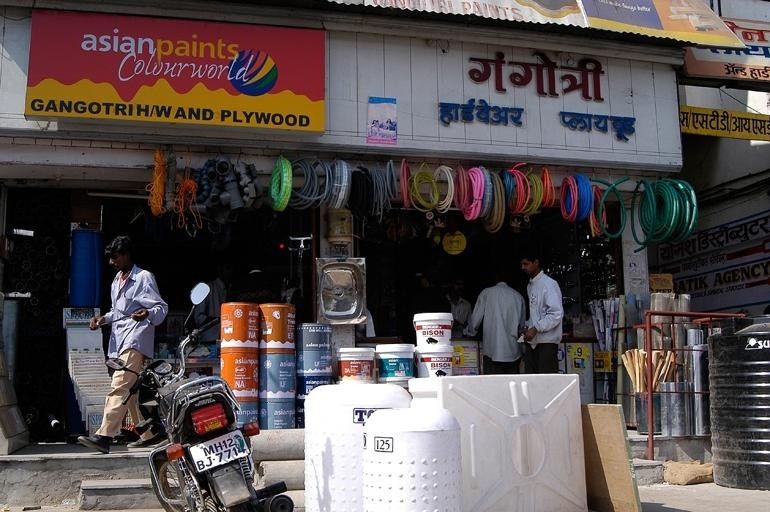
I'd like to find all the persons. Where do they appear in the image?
[194,271,227,345]
[408,247,564,374]
[77,236,168,453]
[234,266,278,303]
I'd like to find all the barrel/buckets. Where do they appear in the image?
[450,338,480,376]
[416,346,454,378]
[219,302,260,354]
[296,322,334,376]
[363,407,461,512]
[375,343,416,378]
[219,354,260,402]
[706,315,770,492]
[235,402,258,430]
[304,385,412,512]
[412,312,454,345]
[259,401,296,431]
[260,353,296,402]
[336,347,375,382]
[376,376,416,400]
[297,376,333,429]
[257,302,296,355]
[634,381,695,437]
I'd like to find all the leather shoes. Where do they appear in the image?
[77,435,110,454]
[126,438,163,447]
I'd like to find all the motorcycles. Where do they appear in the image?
[101,281,298,512]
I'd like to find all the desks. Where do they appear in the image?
[453,337,599,404]
